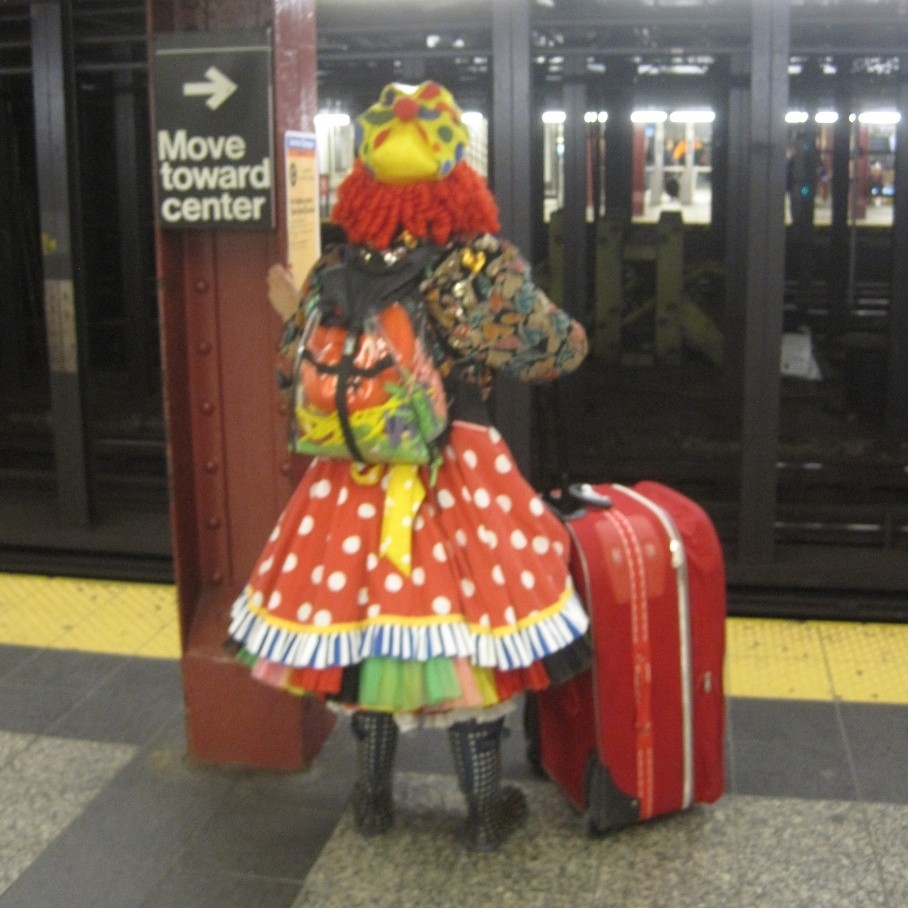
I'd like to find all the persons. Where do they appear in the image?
[259,79,585,860]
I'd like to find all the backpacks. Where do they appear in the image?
[284,227,472,466]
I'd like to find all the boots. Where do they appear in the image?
[348,710,398,829]
[445,725,526,855]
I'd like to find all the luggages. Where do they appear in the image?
[532,381,728,840]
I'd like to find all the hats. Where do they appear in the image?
[331,81,501,247]
